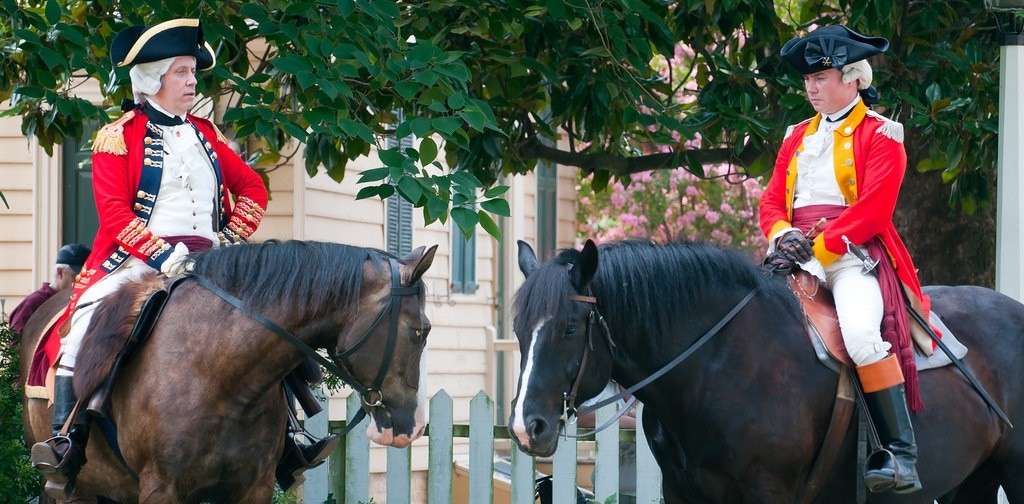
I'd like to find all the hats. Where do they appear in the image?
[109,19,215,72]
[780,25,889,75]
[55,243,91,274]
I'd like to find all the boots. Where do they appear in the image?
[275,422,340,494]
[31,376,93,501]
[855,353,922,495]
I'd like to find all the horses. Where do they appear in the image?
[18,239,438,504]
[508,237,1023,503]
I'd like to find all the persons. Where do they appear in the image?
[31,19,341,504]
[758,24,924,495]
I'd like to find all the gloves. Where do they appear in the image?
[160,242,196,278]
[763,230,815,269]
